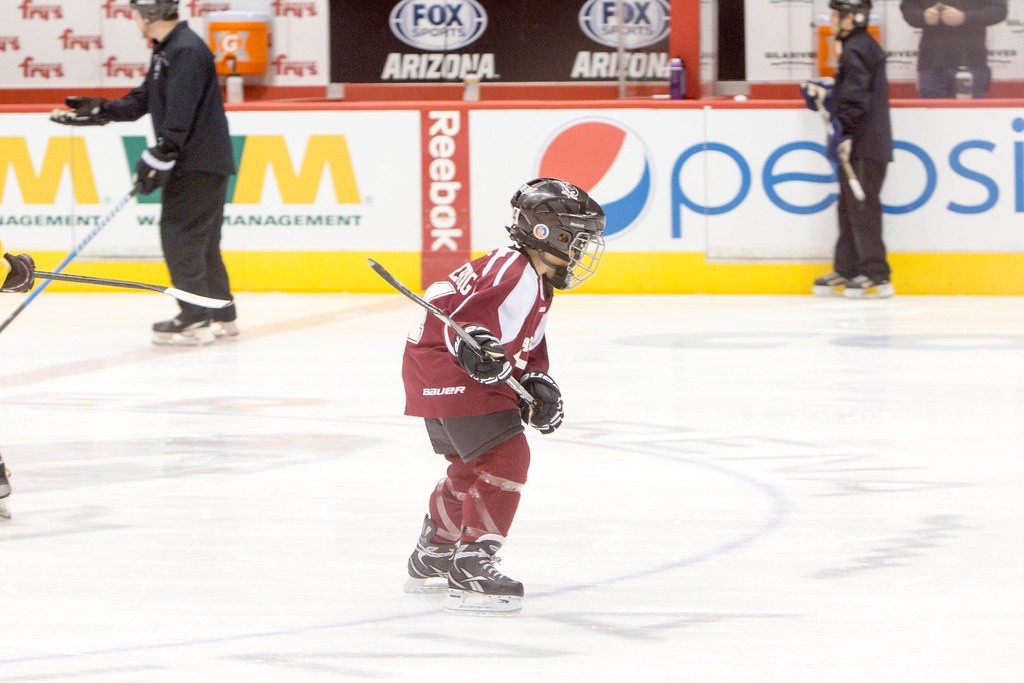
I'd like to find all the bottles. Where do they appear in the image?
[463,68,479,102]
[668,57,688,99]
[953,65,974,100]
[226,72,245,105]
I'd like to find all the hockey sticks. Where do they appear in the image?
[367,256,536,405]
[31,271,234,310]
[0,184,144,334]
[814,96,867,223]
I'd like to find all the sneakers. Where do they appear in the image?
[203,298,237,336]
[814,272,849,295]
[154,312,217,346]
[447,533,525,612]
[1,454,12,519]
[844,276,895,297]
[406,515,457,591]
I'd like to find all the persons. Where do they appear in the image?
[402,178,607,612]
[900,0,1008,99]
[50,0,240,344]
[0,254,36,518]
[800,0,893,299]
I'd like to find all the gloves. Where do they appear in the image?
[49,93,111,126]
[827,118,856,164]
[1,253,36,293]
[516,370,565,433]
[137,139,176,195]
[799,75,836,110]
[454,326,512,386]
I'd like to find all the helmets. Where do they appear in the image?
[829,0,872,41]
[506,178,606,291]
[129,0,182,26]
